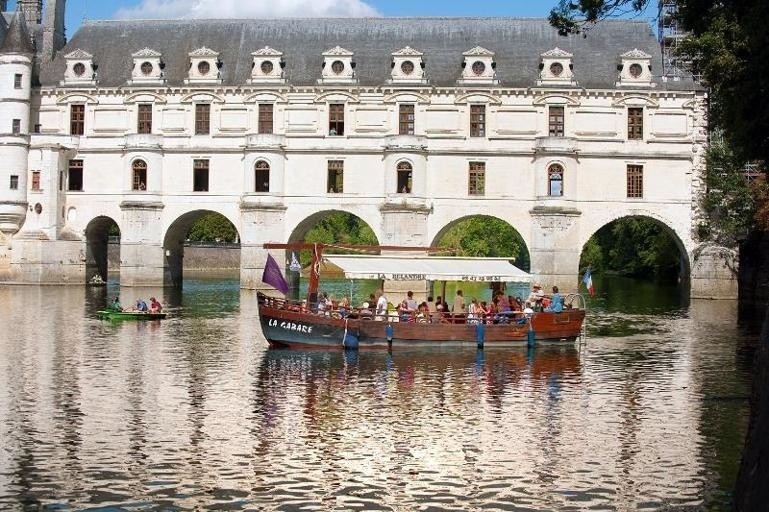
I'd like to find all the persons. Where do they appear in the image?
[92,274,103,282]
[111,297,123,312]
[149,297,162,313]
[316,286,564,323]
[134,297,147,314]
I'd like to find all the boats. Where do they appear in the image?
[96,310,166,321]
[254,242,586,351]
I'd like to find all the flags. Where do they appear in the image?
[262,252,289,294]
[583,267,594,298]
